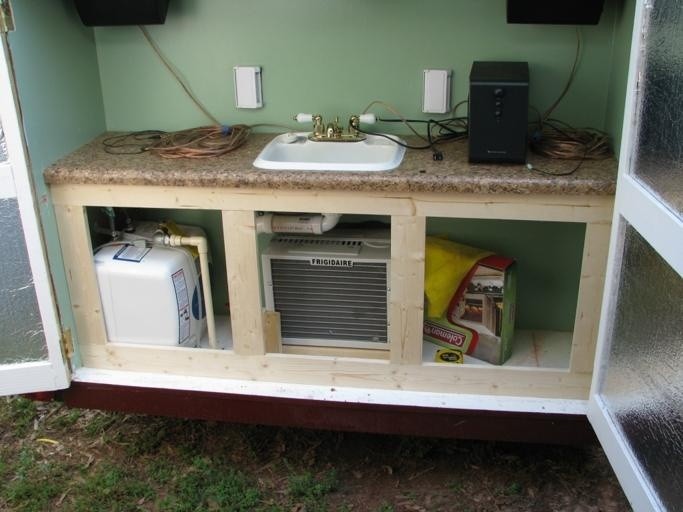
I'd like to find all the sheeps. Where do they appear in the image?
[325,116,342,139]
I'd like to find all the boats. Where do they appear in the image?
[75,0,169,27]
[506,0,603,26]
[467,60,530,164]
